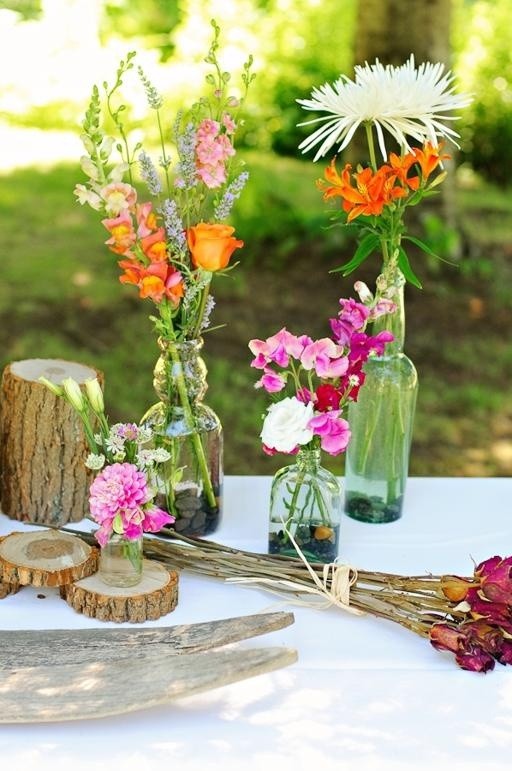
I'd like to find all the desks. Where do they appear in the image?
[1,477,512,759]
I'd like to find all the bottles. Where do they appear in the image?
[135,334,224,539]
[265,447,345,564]
[100,526,146,590]
[344,274,421,525]
